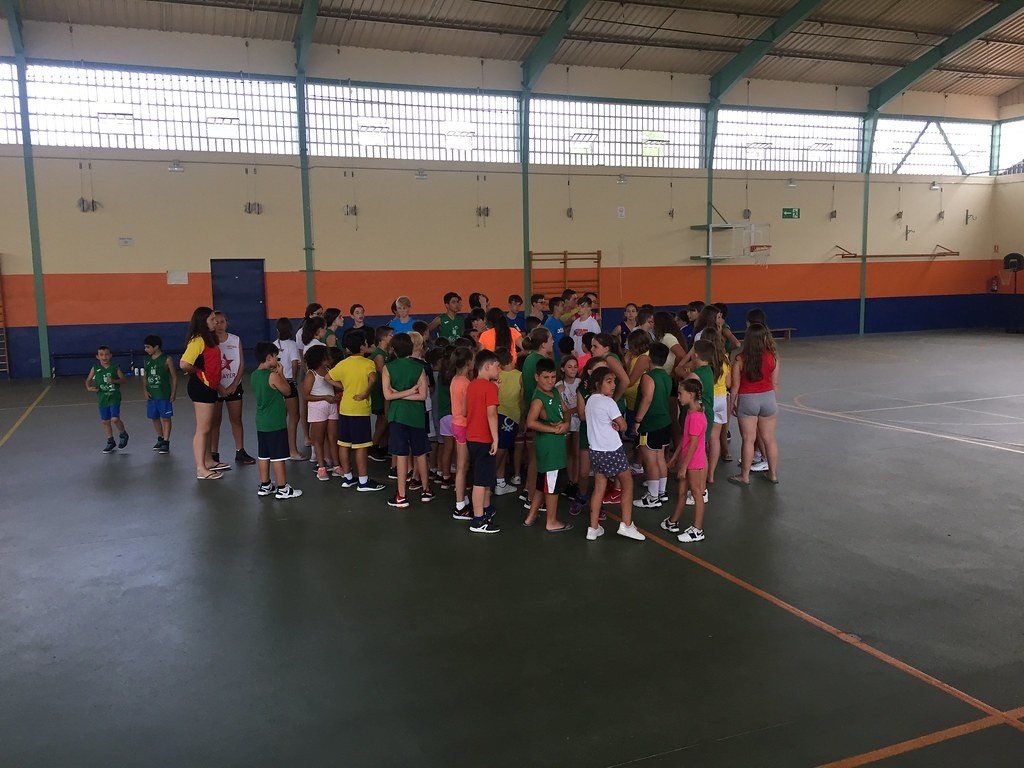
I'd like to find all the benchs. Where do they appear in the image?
[50,349,188,379]
[732,328,796,341]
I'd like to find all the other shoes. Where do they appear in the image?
[726,451,779,487]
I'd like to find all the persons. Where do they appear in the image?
[85,345,129,453]
[584,366,645,541]
[210,310,256,463]
[272,318,308,460]
[730,309,779,487]
[250,344,302,499]
[462,349,502,534]
[522,357,574,532]
[142,335,176,453]
[325,329,385,491]
[180,307,231,479]
[382,333,436,507]
[296,289,740,519]
[661,379,707,542]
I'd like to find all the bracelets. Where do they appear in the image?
[635,420,640,423]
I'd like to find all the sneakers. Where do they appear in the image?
[341,476,359,488]
[367,444,397,461]
[153,435,170,453]
[421,488,437,502]
[483,504,497,518]
[235,449,256,464]
[387,462,522,494]
[356,478,385,491]
[316,467,330,481]
[518,465,709,543]
[103,432,129,454]
[257,482,303,499]
[469,518,502,533]
[387,491,409,508]
[453,506,474,520]
[332,466,353,478]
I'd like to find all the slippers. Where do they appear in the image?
[289,452,309,460]
[196,462,231,479]
